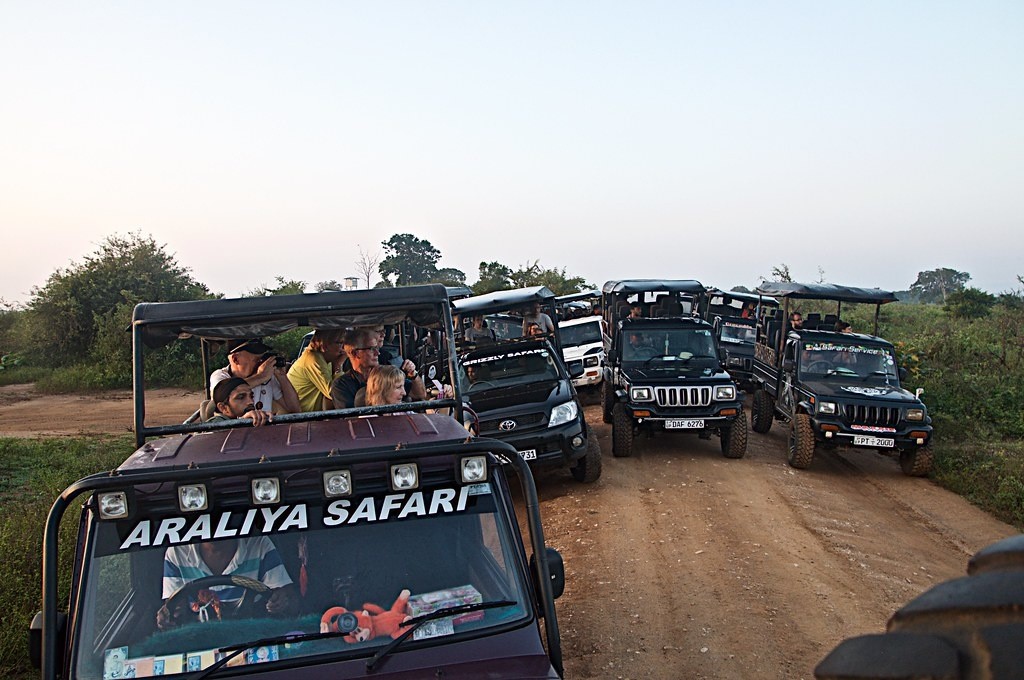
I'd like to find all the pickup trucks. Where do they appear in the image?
[29,281,567,680]
[287,284,602,484]
[554,290,605,398]
[752,282,932,476]
[675,291,781,393]
[603,279,748,458]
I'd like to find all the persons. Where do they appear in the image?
[785,312,856,372]
[465,314,494,342]
[627,302,641,318]
[557,304,602,321]
[468,364,481,382]
[742,303,756,318]
[199,322,454,428]
[630,334,650,355]
[156,535,294,630]
[522,302,555,339]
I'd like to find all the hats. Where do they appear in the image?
[227,335,272,355]
[214,377,249,413]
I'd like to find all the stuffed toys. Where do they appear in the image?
[320,590,413,643]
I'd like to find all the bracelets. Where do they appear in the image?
[407,370,417,380]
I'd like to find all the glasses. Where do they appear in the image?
[375,329,386,336]
[793,318,802,322]
[333,340,343,348]
[355,346,380,353]
[229,336,264,354]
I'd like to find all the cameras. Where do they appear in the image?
[259,353,286,368]
